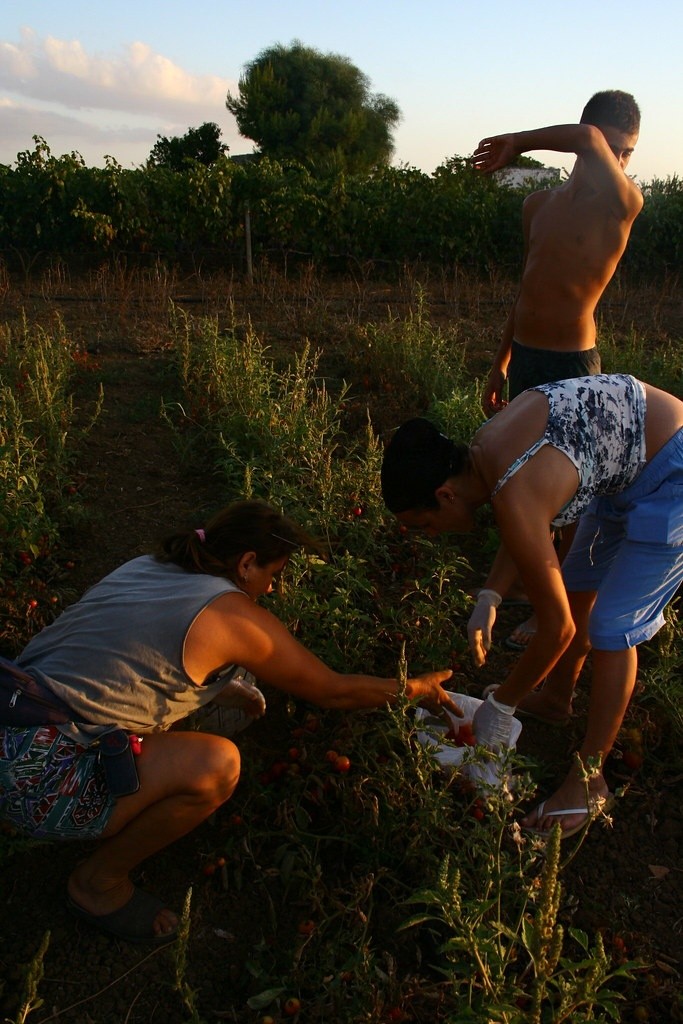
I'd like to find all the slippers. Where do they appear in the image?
[505,621,538,650]
[522,790,616,839]
[482,683,570,728]
[67,888,182,945]
[502,601,533,607]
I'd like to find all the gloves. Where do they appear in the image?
[471,691,517,755]
[467,589,502,668]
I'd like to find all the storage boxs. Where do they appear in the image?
[414,690,486,775]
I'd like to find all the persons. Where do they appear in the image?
[473,93,644,651]
[0,502,464,947]
[381,375,683,840]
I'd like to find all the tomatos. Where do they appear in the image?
[0,332,655,978]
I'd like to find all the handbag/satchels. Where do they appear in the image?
[176,664,257,736]
[415,689,522,802]
[0,657,80,726]
